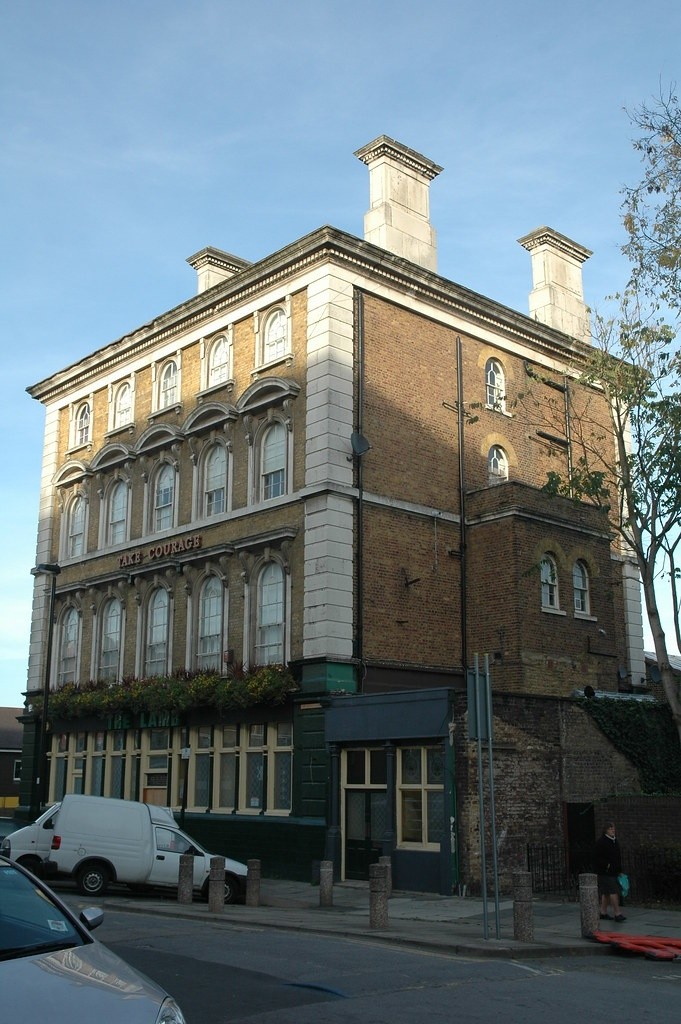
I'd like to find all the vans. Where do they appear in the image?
[0,800,176,881]
[47,793,249,900]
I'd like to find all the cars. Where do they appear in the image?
[0,858,188,1024]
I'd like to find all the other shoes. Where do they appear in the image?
[599,913,615,920]
[614,914,626,922]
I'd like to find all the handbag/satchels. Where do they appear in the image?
[618,874,630,897]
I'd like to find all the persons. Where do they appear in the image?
[597,822,627,922]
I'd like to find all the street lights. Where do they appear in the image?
[29,563,61,820]
